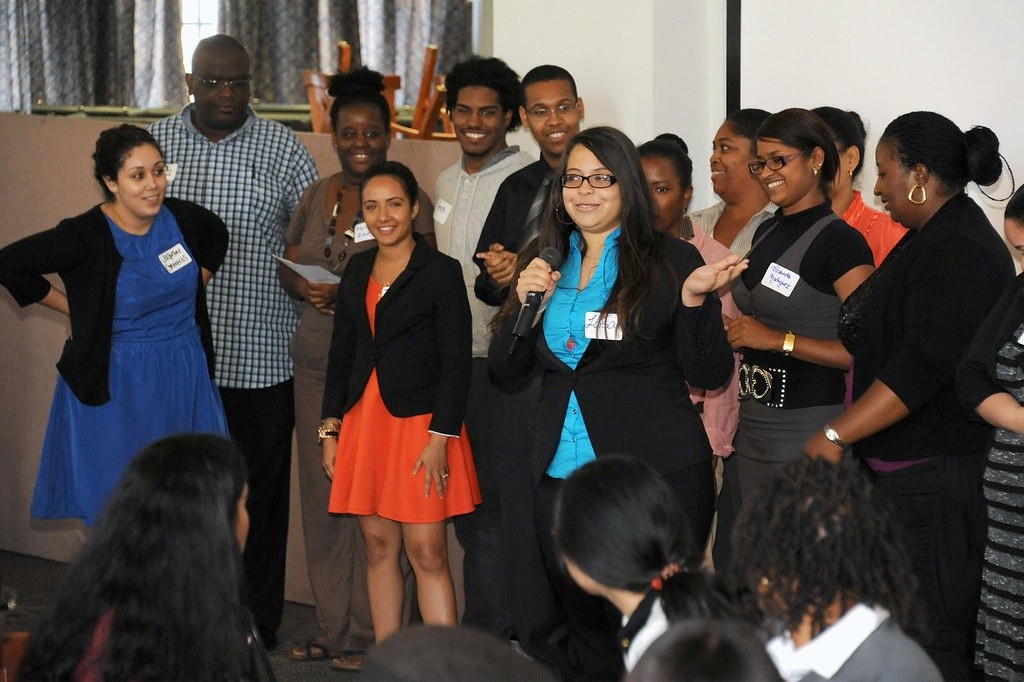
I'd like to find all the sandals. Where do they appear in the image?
[287,636,340,661]
[329,649,370,673]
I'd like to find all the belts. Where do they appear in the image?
[735,358,846,409]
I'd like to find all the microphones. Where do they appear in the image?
[509,246,562,356]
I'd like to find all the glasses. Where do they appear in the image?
[747,149,807,176]
[559,173,618,188]
[523,99,579,120]
[188,72,253,96]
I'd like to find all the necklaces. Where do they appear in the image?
[325,179,363,265]
[377,250,407,302]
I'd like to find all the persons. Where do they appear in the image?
[731,457,943,682]
[472,65,585,662]
[320,160,483,644]
[550,454,764,672]
[144,34,320,656]
[0,124,228,532]
[640,106,1024,682]
[18,433,252,682]
[628,617,776,682]
[487,124,749,682]
[433,56,538,645]
[278,66,436,670]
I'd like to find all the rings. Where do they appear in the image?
[441,475,446,478]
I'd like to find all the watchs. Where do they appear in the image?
[317,427,339,443]
[823,424,849,451]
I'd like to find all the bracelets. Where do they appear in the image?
[782,330,795,356]
